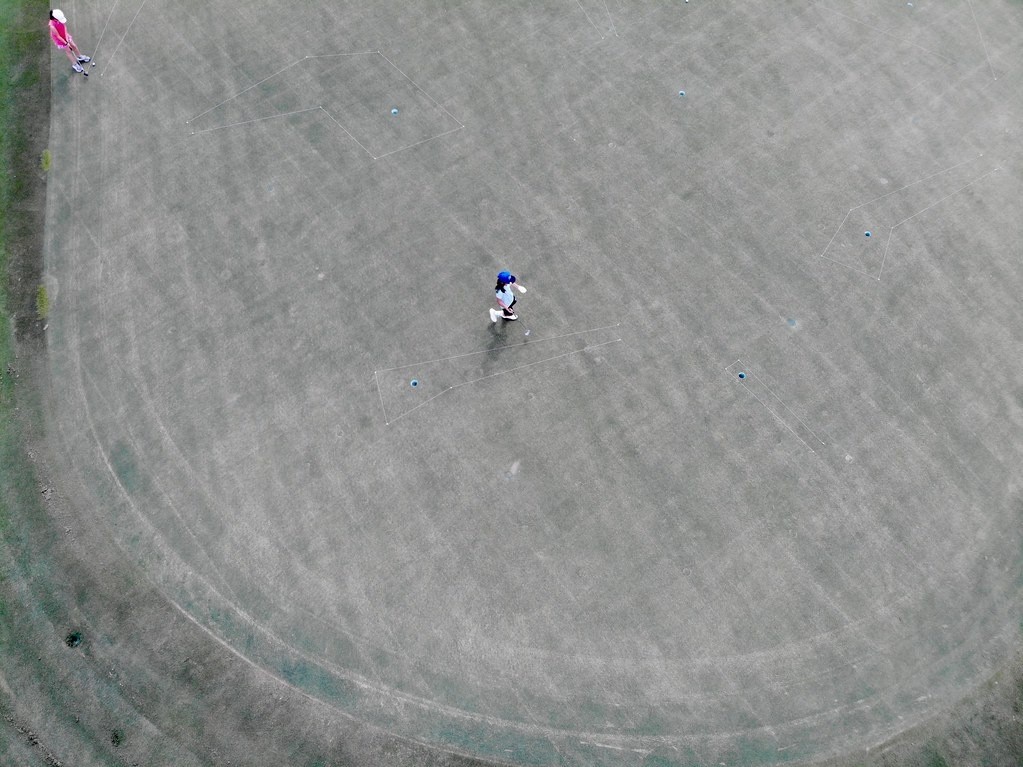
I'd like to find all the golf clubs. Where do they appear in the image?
[66,41,88,76]
[510,308,530,336]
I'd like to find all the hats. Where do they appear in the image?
[498,271,516,284]
[52,8,66,23]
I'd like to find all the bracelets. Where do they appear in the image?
[66,42,68,45]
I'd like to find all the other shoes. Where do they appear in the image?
[78,55,91,63]
[72,64,83,72]
[489,308,497,322]
[502,314,518,320]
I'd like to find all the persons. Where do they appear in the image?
[489,271,527,322]
[49,10,91,72]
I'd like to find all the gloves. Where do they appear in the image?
[517,285,527,294]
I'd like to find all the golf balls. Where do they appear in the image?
[93,63,95,65]
